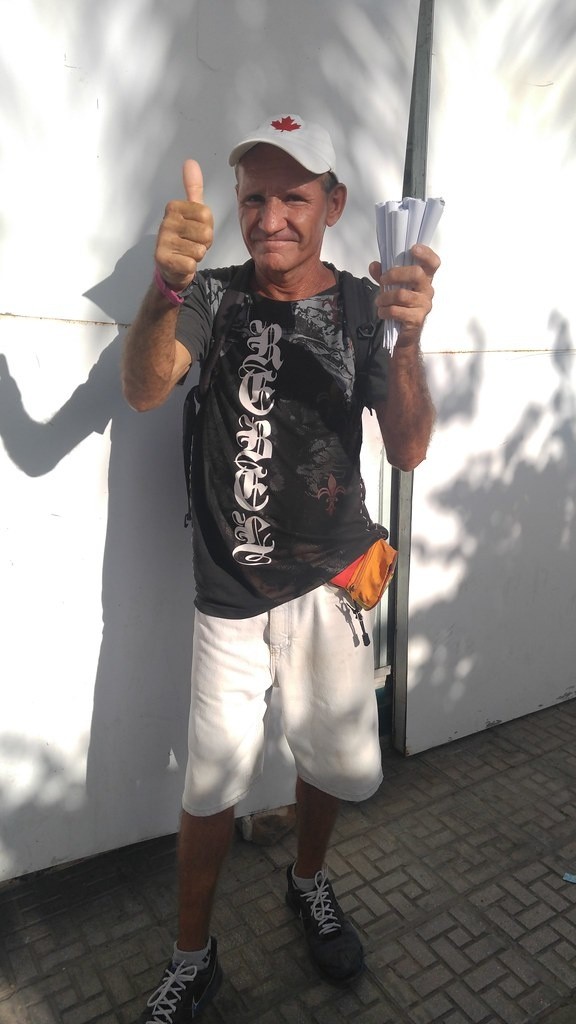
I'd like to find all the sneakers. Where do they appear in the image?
[136,935,224,1024]
[286,859,365,987]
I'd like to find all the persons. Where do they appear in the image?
[119,113,442,1024]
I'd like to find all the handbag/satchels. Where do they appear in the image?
[328,537,400,647]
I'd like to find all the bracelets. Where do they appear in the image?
[154,267,200,307]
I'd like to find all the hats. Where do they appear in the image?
[229,113,336,175]
